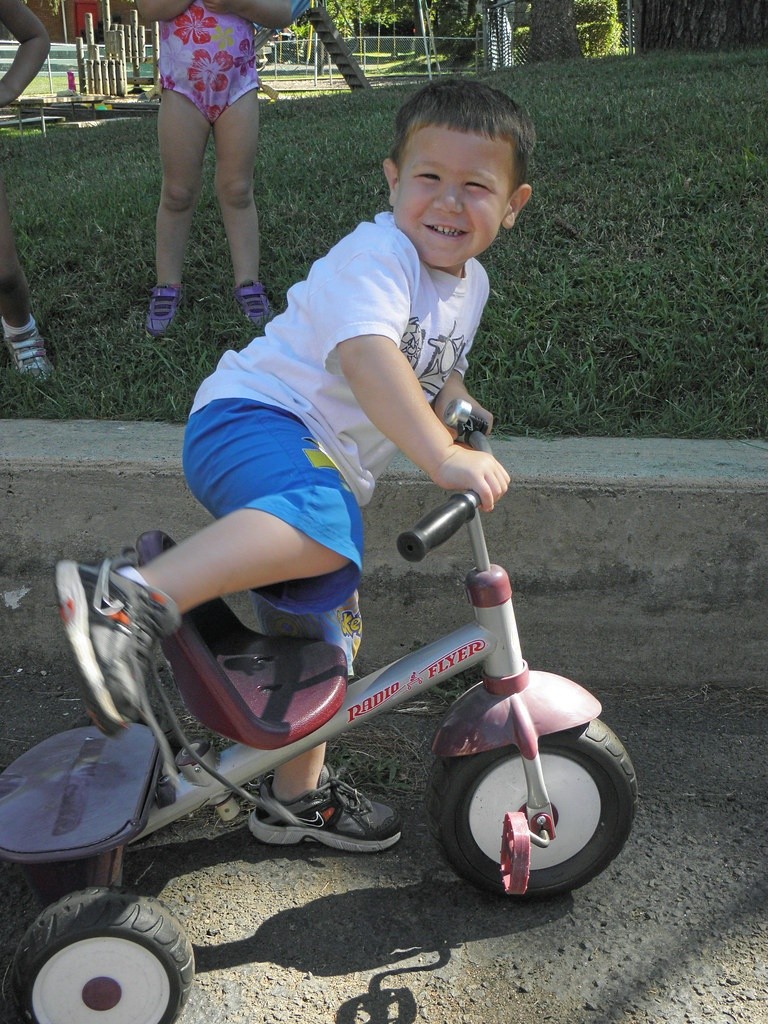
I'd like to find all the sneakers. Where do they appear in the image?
[144,282,183,339]
[232,279,278,328]
[3,331,56,385]
[246,762,403,854]
[55,552,182,737]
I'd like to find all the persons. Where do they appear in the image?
[135,0,292,340]
[55,81,532,852]
[0,0,52,383]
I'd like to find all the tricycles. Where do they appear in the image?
[0,399,639,1024]
